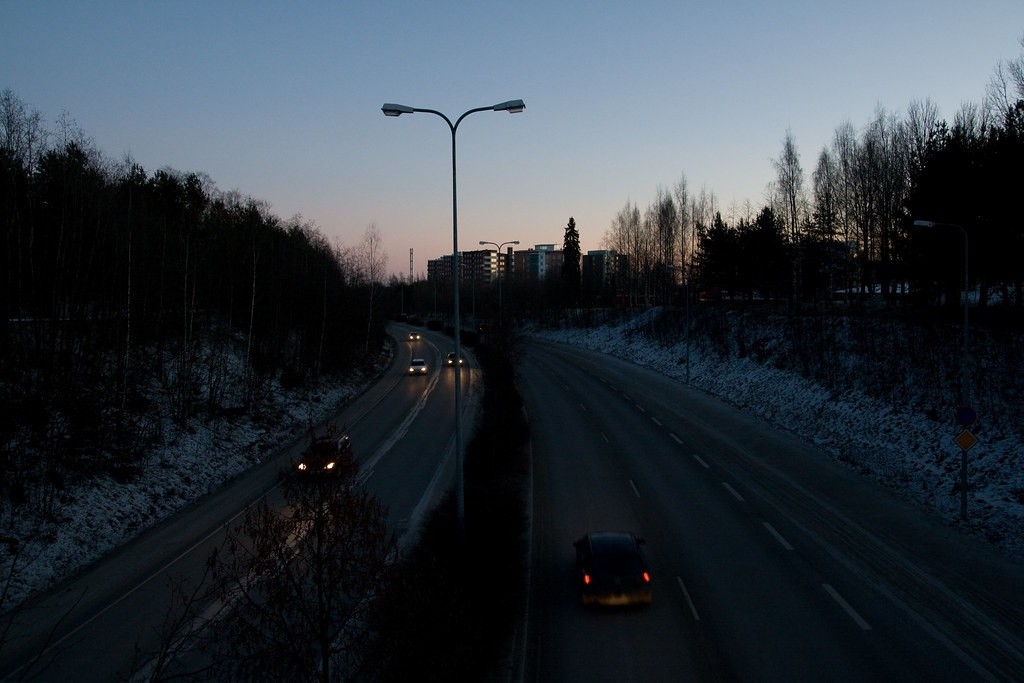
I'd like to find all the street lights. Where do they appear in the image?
[459,263,484,332]
[479,241,519,326]
[427,274,436,320]
[914,219,969,518]
[382,100,525,522]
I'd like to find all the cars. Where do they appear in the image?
[408,358,428,375]
[408,332,421,341]
[446,351,464,366]
[572,531,654,615]
[295,432,353,483]
